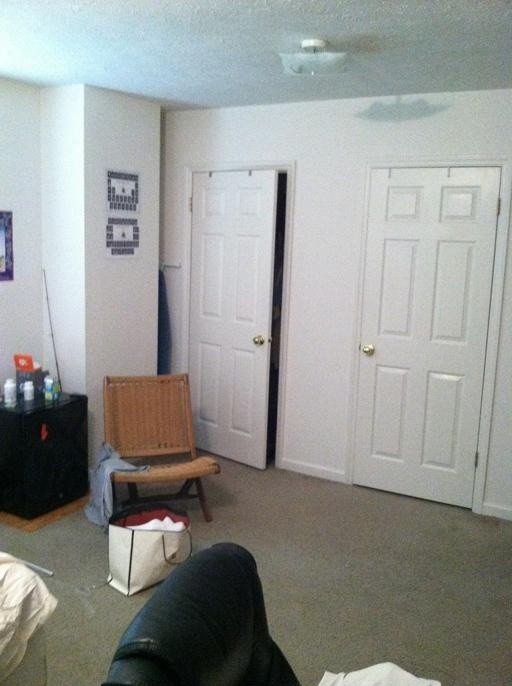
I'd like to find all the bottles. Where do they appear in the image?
[3,379,17,404]
[24,380,34,401]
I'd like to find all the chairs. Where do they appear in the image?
[96,543,299,686]
[101,375,221,524]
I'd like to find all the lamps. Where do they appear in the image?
[282,39,352,78]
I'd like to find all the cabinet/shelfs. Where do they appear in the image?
[0,392,89,521]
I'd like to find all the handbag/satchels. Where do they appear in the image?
[106,501,194,598]
